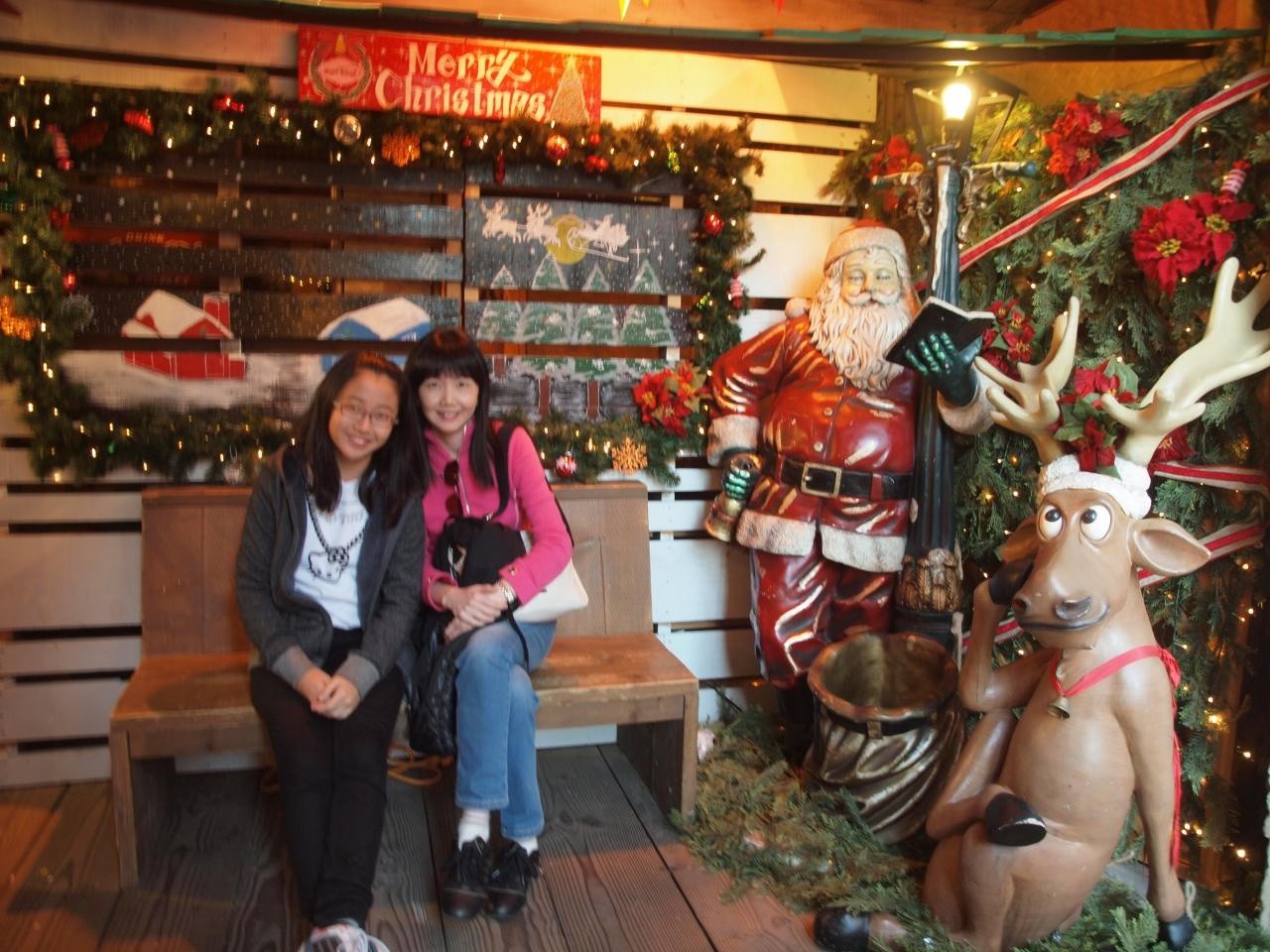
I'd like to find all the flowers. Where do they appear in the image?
[978,300,1035,383]
[628,364,703,438]
[866,135,923,215]
[1130,194,1251,294]
[1046,357,1193,466]
[1039,102,1131,183]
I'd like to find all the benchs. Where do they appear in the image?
[110,480,699,891]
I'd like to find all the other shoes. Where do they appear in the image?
[488,836,542,922]
[443,823,494,921]
[297,924,387,952]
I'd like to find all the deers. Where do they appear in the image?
[812,258,1269,952]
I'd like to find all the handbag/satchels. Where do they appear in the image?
[502,529,590,624]
[432,516,527,589]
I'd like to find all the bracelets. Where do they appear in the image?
[496,578,514,606]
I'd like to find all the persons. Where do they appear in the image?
[235,350,425,952]
[708,215,1003,777]
[402,330,575,919]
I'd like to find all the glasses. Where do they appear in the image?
[444,459,464,519]
[331,399,400,426]
